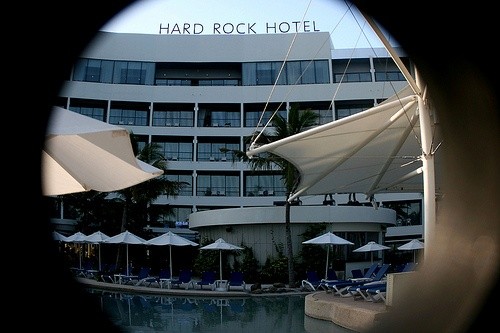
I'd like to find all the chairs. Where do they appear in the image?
[226,272,246,291]
[169,270,194,289]
[144,269,170,287]
[124,269,149,285]
[196,270,215,290]
[302,261,416,304]
[75,262,132,284]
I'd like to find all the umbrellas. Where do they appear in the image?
[54,231,67,240]
[42,107,163,196]
[100,230,147,276]
[302,231,354,280]
[84,231,111,269]
[199,237,245,280]
[146,232,198,279]
[351,241,391,266]
[62,231,98,268]
[397,238,424,264]
